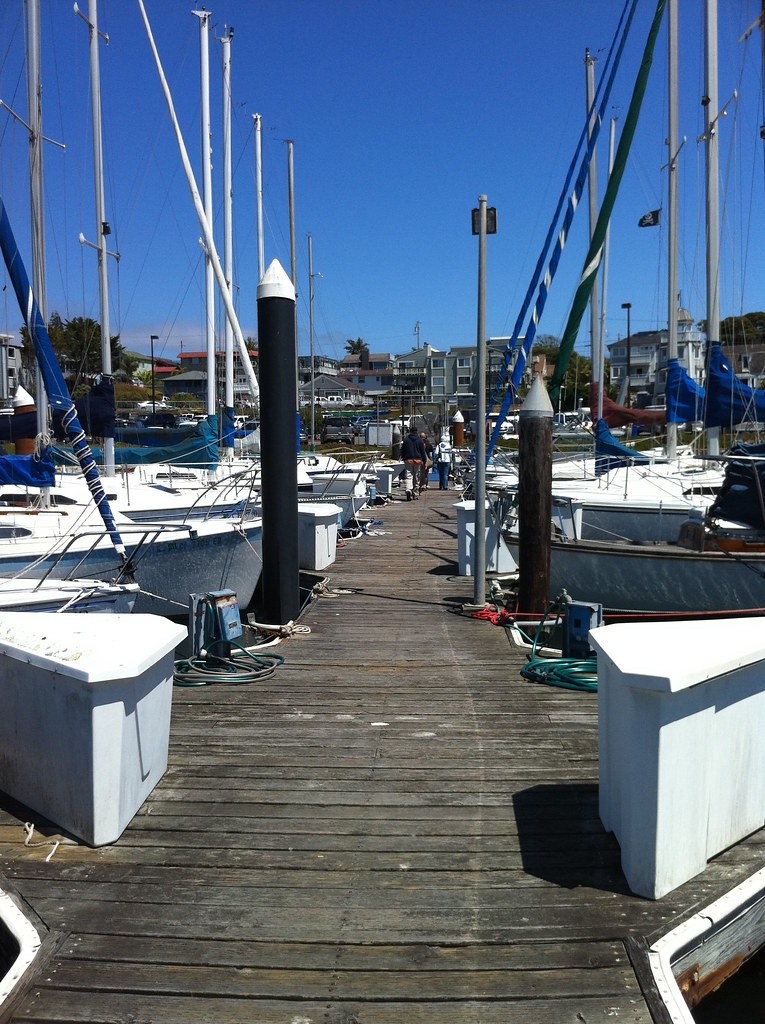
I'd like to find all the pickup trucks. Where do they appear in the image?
[470,412,515,437]
[299,396,354,411]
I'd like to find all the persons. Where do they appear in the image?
[401,427,453,501]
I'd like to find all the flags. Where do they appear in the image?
[638,209,660,227]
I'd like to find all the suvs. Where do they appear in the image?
[321,416,356,443]
[299,423,310,444]
[390,414,423,428]
[450,411,480,429]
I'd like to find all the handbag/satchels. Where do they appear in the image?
[434,444,441,463]
[425,451,434,470]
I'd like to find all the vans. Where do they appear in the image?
[1,4,375,630]
[115,412,132,426]
[179,415,208,429]
[130,412,148,419]
[233,416,249,427]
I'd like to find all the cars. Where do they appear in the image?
[553,411,593,430]
[505,410,520,422]
[355,417,388,435]
[136,400,170,411]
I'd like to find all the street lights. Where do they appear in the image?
[559,384,565,415]
[149,333,158,416]
[620,303,631,410]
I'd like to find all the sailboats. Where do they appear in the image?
[458,0,764,615]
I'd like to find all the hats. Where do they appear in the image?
[441,435,447,442]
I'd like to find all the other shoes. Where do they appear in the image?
[444,488,448,490]
[413,492,419,500]
[439,487,442,490]
[420,493,423,495]
[421,485,427,491]
[427,486,430,488]
[406,490,411,501]
